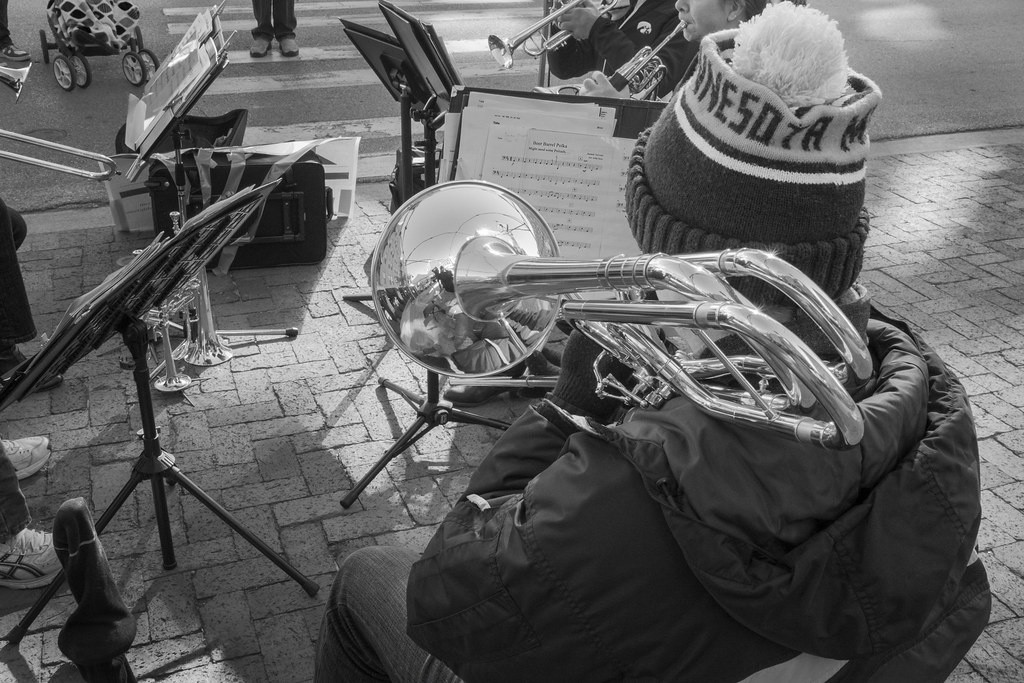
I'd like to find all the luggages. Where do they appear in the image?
[144,151,333,271]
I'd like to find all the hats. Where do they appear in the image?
[624,2,882,304]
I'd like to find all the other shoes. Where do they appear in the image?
[0,343,63,392]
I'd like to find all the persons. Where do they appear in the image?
[0,0,31,66]
[0,200,65,590]
[250,0,299,57]
[315,0,992,683]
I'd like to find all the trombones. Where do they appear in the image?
[369,179,875,447]
[0,62,117,184]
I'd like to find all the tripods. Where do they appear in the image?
[1,177,318,649]
[341,0,514,506]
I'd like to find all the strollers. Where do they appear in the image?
[39,0,162,92]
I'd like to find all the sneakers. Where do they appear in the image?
[249,36,271,58]
[279,37,300,57]
[0,44,32,62]
[0,528,65,589]
[1,435,52,480]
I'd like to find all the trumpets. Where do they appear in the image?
[166,210,236,365]
[487,0,617,72]
[131,249,186,393]
[535,20,689,100]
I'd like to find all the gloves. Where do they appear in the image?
[551,330,637,425]
